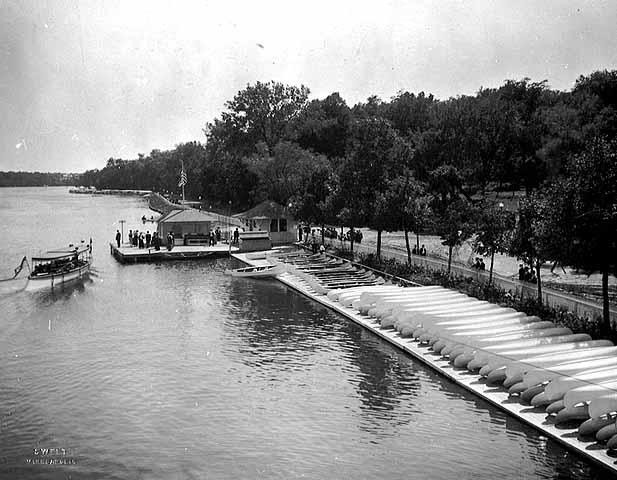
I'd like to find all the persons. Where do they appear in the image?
[34,257,84,275]
[115,229,121,248]
[479,258,485,272]
[518,263,524,280]
[523,267,531,282]
[412,244,417,255]
[128,229,175,251]
[232,227,240,245]
[296,223,363,256]
[470,257,481,270]
[528,270,538,285]
[215,226,222,246]
[419,244,427,256]
[208,227,216,247]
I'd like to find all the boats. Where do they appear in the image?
[223,263,286,278]
[24,237,95,293]
[245,248,617,465]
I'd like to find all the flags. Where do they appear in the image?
[177,160,188,188]
